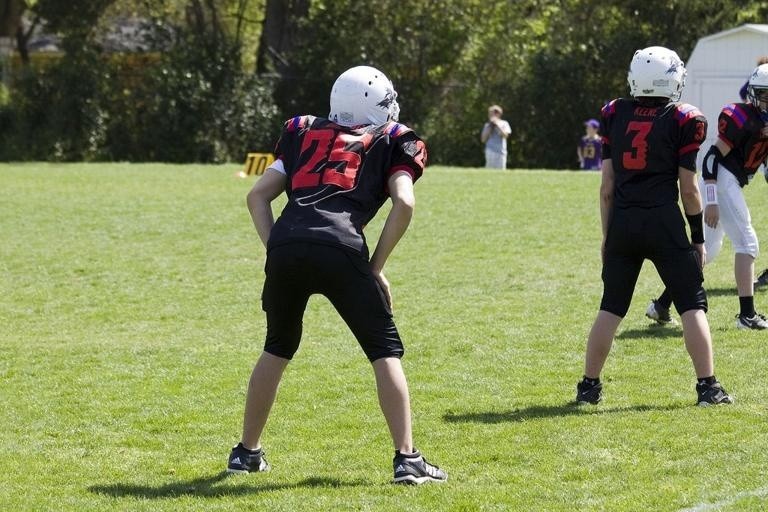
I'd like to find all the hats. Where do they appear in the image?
[582,119,600,128]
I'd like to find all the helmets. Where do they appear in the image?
[627,46,687,101]
[747,63,768,113]
[328,66,400,127]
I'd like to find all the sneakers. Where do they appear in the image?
[393,446,447,484]
[228,443,271,474]
[696,382,734,407]
[735,312,768,329]
[576,379,604,406]
[645,299,678,326]
[751,270,768,291]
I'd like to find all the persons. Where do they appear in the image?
[576,119,603,171]
[574,46,736,405]
[220,62,450,486]
[644,63,767,331]
[480,104,510,169]
[739,57,767,294]
